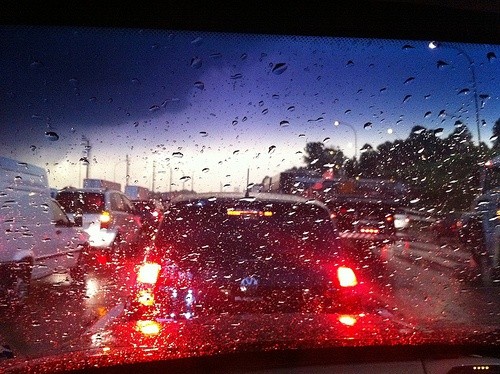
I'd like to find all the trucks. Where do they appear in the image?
[1,158,90,320]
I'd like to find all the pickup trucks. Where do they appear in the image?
[280,171,401,252]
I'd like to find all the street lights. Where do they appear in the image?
[335,121,358,164]
[429,42,481,158]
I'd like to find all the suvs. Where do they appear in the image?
[126,193,378,356]
[54,188,144,265]
